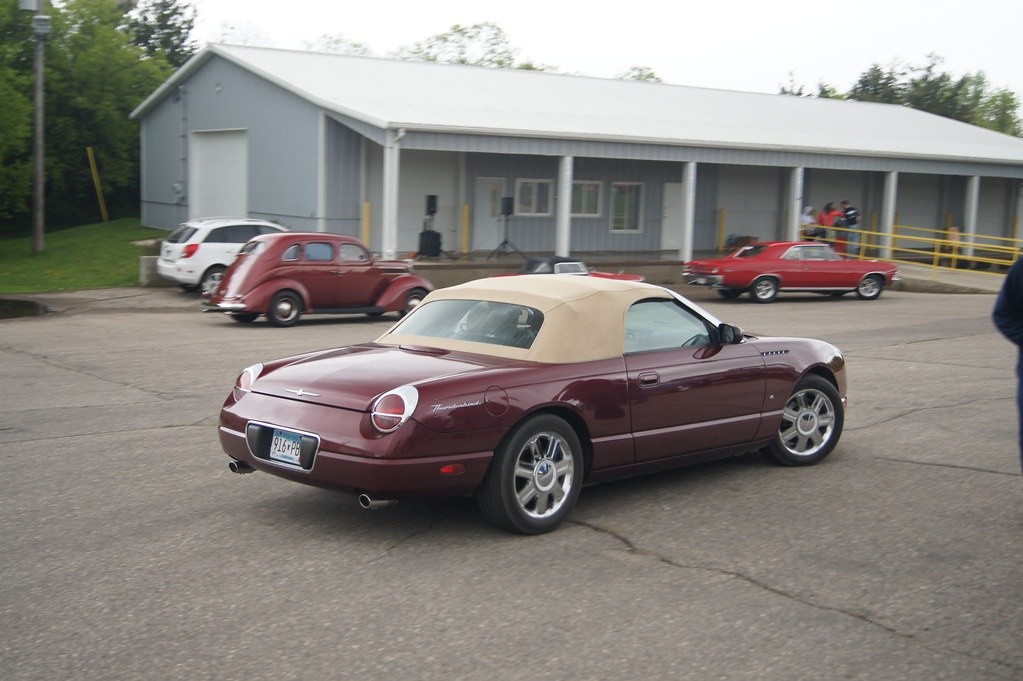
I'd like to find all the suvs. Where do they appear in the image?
[156,214,290,299]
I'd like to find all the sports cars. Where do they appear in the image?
[219,274,851,538]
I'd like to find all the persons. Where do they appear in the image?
[991,252,1023,470]
[801,199,862,259]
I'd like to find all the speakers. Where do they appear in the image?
[426,195,437,214]
[501,197,513,214]
[420,231,441,257]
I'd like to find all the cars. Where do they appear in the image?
[201,231,437,328]
[682,239,902,304]
[516,258,642,289]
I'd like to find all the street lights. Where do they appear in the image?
[29,11,53,256]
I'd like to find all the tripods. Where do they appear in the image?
[415,214,458,260]
[488,214,529,260]
